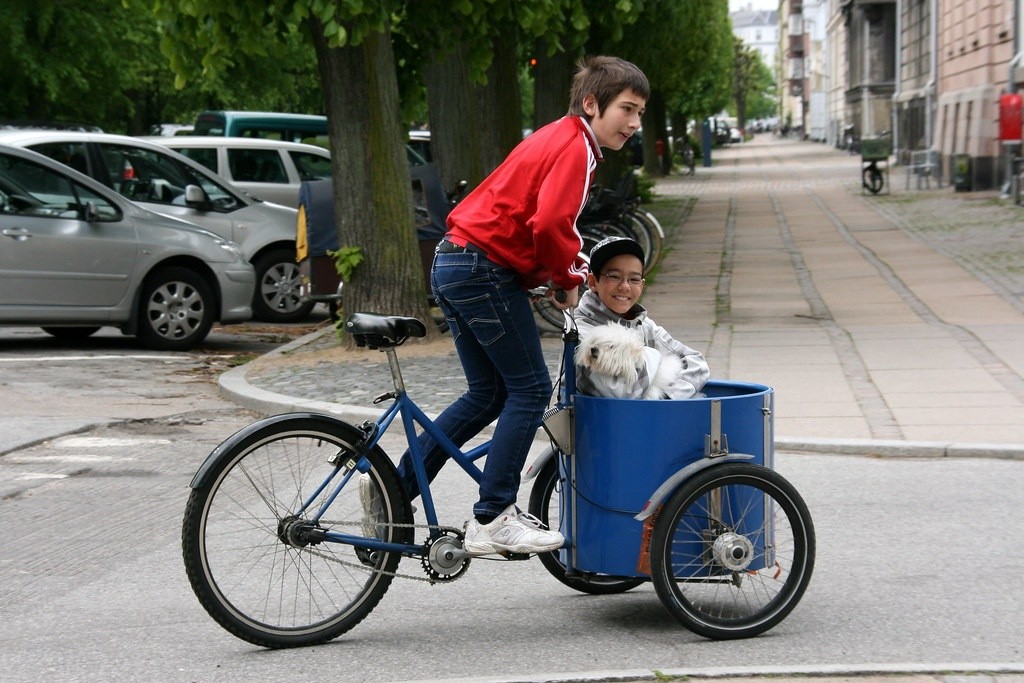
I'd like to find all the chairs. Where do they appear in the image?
[905,145,943,190]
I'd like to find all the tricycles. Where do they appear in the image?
[181,283,817,649]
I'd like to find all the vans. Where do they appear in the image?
[122,136,334,204]
[190,113,329,178]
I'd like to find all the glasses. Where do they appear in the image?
[594,271,646,287]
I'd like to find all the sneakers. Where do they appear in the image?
[462,505,565,555]
[358,473,418,541]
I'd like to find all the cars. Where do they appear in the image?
[407,132,436,168]
[1,145,257,346]
[710,116,741,143]
[125,123,224,173]
[0,128,316,323]
[746,118,780,133]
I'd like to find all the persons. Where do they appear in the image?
[556,236,711,400]
[357,56,651,555]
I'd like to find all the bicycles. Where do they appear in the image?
[671,135,696,176]
[853,130,892,194]
[410,170,665,329]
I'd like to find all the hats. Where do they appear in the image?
[589,236,645,273]
